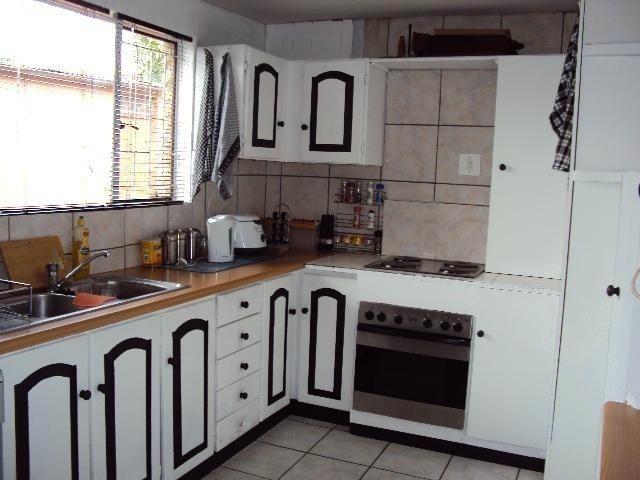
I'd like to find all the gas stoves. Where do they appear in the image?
[364,255,485,279]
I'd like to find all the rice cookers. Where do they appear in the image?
[233,213,268,257]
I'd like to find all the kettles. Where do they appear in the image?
[205,215,239,263]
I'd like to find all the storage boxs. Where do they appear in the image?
[411,28,525,57]
[290,219,319,251]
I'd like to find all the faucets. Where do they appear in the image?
[48,249,110,291]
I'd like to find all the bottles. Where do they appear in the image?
[367,210,375,230]
[353,207,362,229]
[72,216,91,280]
[340,180,384,203]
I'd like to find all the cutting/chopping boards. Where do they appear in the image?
[0,235,72,291]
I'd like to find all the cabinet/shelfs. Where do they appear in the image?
[160,293,217,480]
[465,287,565,474]
[262,273,294,434]
[215,283,263,469]
[295,268,358,427]
[333,193,383,255]
[0,310,160,480]
[192,44,387,166]
[484,55,569,279]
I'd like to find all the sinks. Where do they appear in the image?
[0,292,103,322]
[65,277,191,303]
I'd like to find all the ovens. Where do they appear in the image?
[353,299,474,431]
